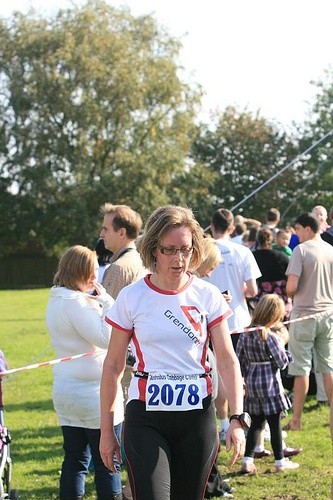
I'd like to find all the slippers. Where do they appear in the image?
[282,420,302,431]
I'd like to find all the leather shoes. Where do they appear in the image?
[253,448,272,458]
[283,447,302,456]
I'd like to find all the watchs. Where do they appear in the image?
[229,412,252,429]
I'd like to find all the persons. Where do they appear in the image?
[201,206,332,473]
[89,205,151,301]
[46,245,124,500]
[99,206,246,500]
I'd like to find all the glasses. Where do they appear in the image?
[102,224,122,233]
[158,243,193,255]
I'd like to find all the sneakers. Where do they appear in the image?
[264,430,287,440]
[241,462,260,472]
[275,458,299,471]
[219,424,230,441]
